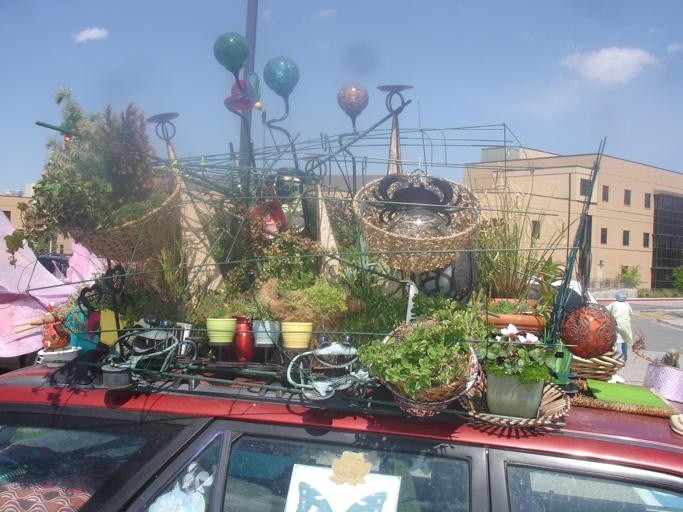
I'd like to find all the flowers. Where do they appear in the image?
[479,324,557,383]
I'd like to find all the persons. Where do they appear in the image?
[606,291,633,362]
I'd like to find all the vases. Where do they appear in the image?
[487,373,545,419]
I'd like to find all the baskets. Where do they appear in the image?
[571,345,624,381]
[70,166,181,262]
[353,174,478,271]
[459,375,570,426]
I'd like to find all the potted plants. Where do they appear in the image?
[206,291,314,350]
[487,298,554,335]
[6,88,181,263]
[357,288,478,418]
